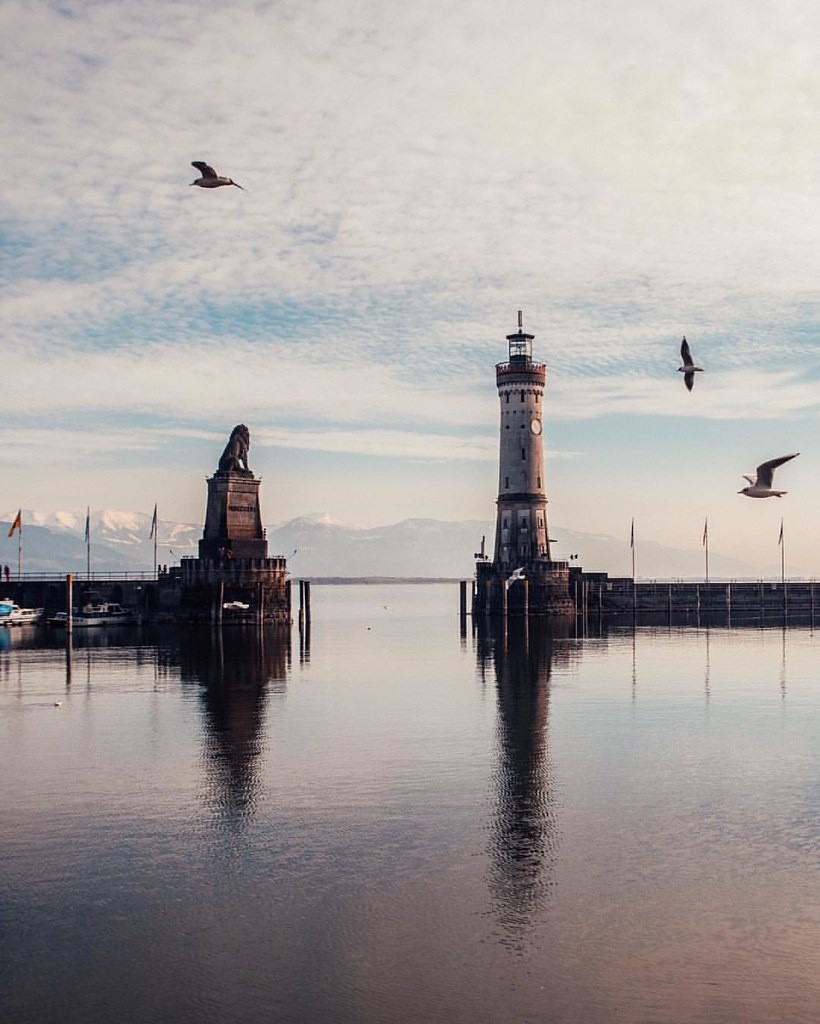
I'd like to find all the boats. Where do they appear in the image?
[47,601,137,627]
[0,596,44,627]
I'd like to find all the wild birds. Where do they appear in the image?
[675,334,706,393]
[188,161,245,193]
[223,601,250,611]
[736,451,802,499]
[54,699,63,707]
[505,567,526,590]
